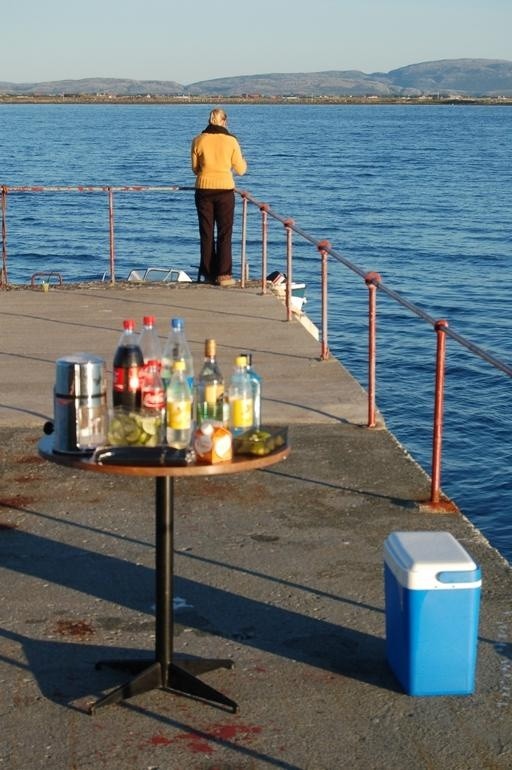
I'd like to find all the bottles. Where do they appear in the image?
[141,360,166,443]
[165,363,192,449]
[162,318,193,420]
[196,339,224,427]
[113,320,142,411]
[222,356,254,440]
[242,353,262,431]
[139,315,161,372]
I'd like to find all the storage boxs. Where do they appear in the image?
[382,530,485,696]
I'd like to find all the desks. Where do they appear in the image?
[36,418,291,715]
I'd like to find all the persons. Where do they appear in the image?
[191,110,246,284]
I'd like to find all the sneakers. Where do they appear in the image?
[215,274,236,286]
